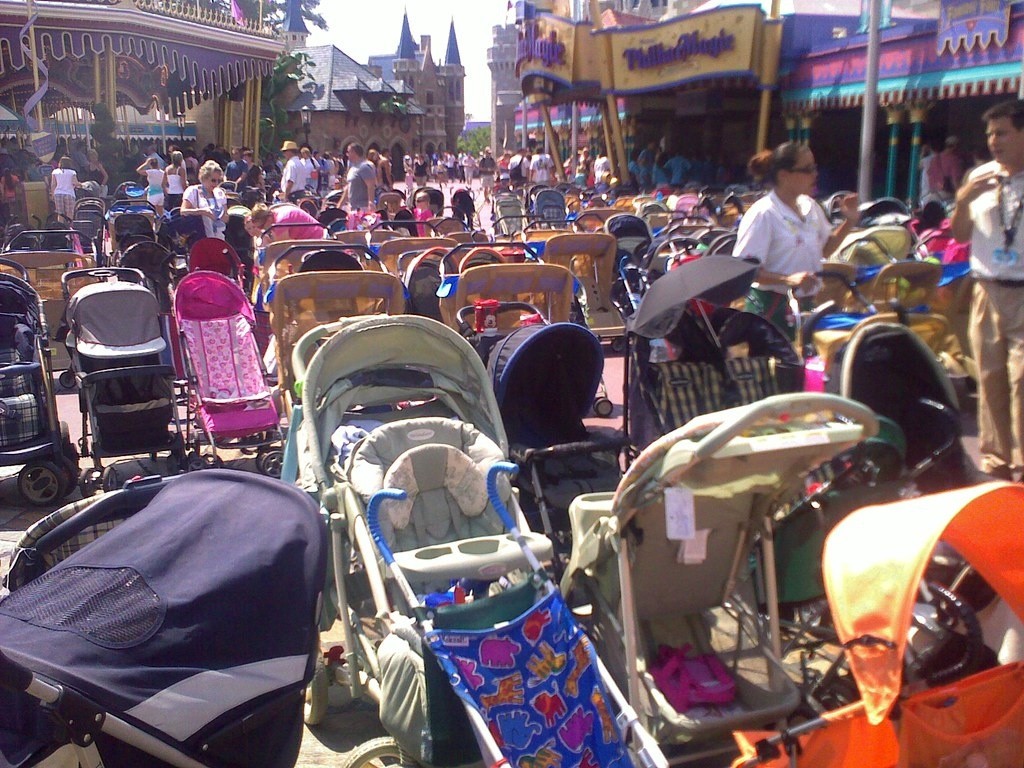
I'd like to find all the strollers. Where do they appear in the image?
[0,176,1024,768]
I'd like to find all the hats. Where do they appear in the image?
[280,141,297,151]
[945,136,961,146]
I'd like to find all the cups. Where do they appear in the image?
[476,305,498,337]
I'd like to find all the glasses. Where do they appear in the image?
[788,162,817,174]
[283,150,288,152]
[206,177,221,183]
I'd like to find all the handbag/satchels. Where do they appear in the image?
[587,174,594,189]
[510,166,522,179]
[650,644,734,712]
[564,168,571,175]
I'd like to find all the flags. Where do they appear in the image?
[231,0,245,27]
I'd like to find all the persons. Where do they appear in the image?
[0,140,731,246]
[917,135,992,209]
[732,142,860,340]
[951,100,1024,483]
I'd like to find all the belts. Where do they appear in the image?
[997,280,1024,287]
